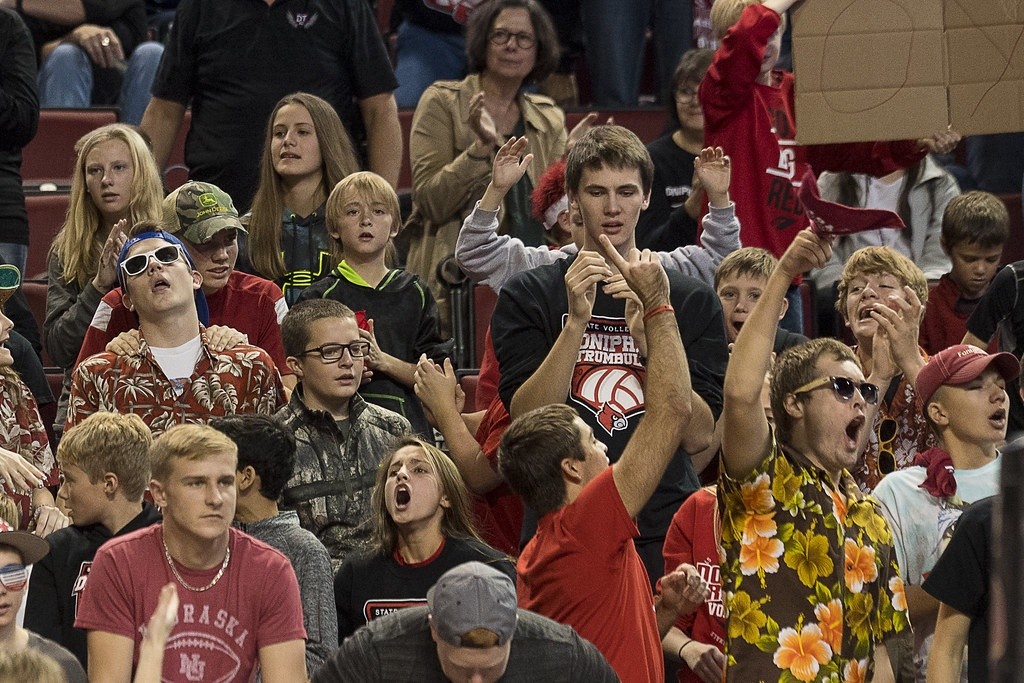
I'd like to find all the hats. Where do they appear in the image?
[426,560,517,648]
[916,344,1020,416]
[0,517,50,565]
[116,229,209,327]
[161,180,249,244]
[797,162,906,235]
[0,264,20,305]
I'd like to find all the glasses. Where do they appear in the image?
[119,243,191,276]
[793,374,881,404]
[0,562,29,592]
[294,340,370,360]
[488,28,537,49]
[671,84,698,104]
[877,416,898,477]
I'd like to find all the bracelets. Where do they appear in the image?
[678,639,694,660]
[642,304,674,323]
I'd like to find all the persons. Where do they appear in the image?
[0,0,1024,683]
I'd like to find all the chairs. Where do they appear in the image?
[398,109,668,558]
[21,105,191,394]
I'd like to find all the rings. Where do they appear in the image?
[100,37,110,47]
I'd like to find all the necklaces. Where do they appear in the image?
[162,536,230,592]
